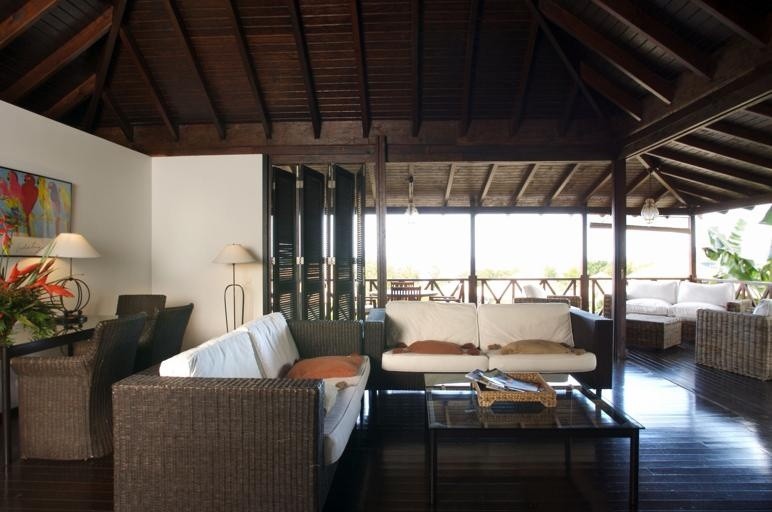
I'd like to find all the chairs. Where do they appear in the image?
[9,293,198,470]
[352,278,465,321]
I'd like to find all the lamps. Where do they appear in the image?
[210,240,261,334]
[640,166,660,226]
[32,228,101,324]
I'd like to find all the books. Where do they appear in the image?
[464,368,546,393]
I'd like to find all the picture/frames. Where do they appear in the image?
[0,164,74,261]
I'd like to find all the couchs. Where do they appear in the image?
[355,300,617,432]
[102,313,373,511]
[508,277,772,385]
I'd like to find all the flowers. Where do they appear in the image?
[0,208,77,350]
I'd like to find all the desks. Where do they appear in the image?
[1,310,120,367]
